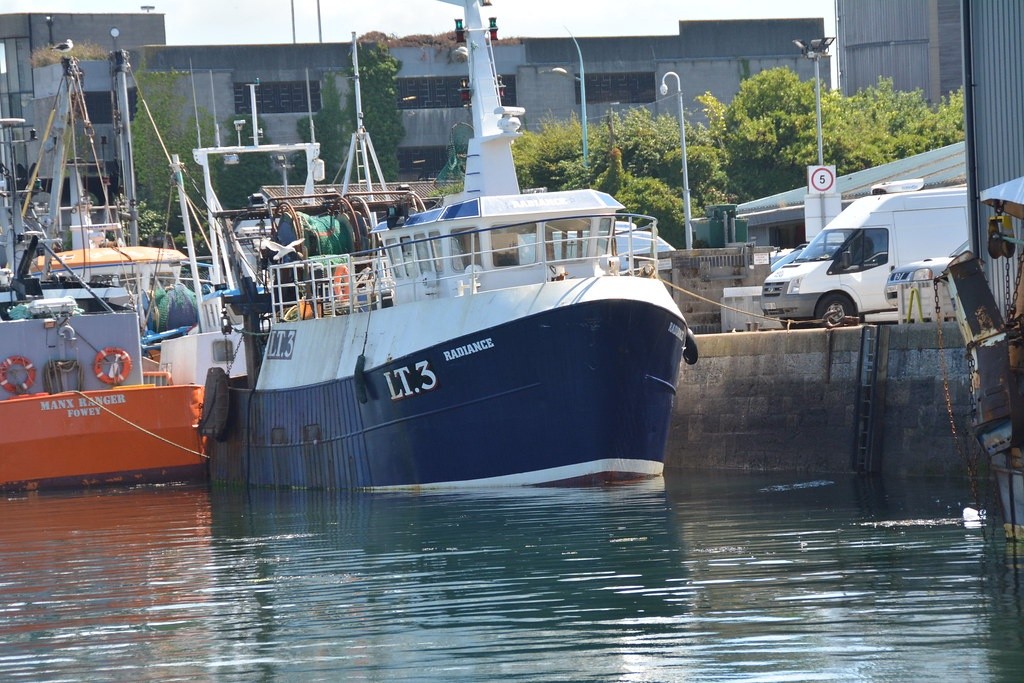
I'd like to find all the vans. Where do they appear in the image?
[760,178,968,329]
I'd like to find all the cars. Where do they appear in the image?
[882,239,969,308]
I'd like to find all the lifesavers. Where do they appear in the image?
[683,327,699,365]
[203,367,229,442]
[0,356,35,393]
[93,346,131,386]
[334,264,350,302]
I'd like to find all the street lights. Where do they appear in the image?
[659,70,693,249]
[794,37,837,229]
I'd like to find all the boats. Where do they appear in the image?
[1,47,205,480]
[149,0,699,493]
